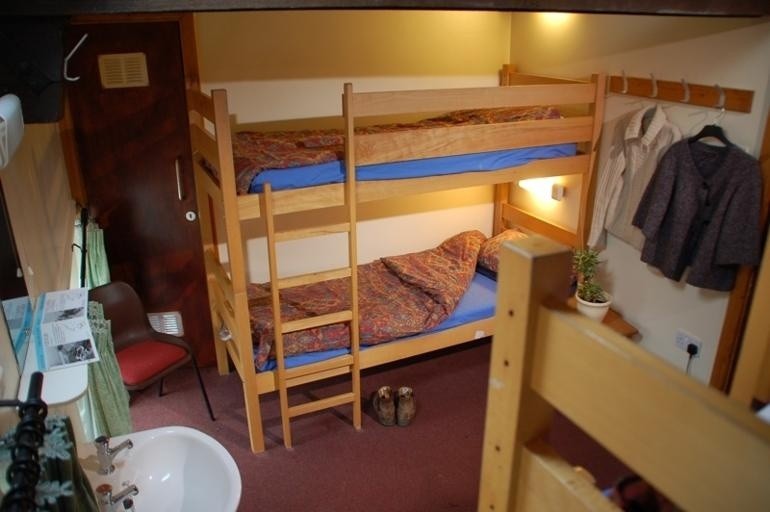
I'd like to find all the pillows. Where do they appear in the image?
[474,226,529,281]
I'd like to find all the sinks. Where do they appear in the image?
[88,426,242,512]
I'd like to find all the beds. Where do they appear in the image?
[181,60,606,450]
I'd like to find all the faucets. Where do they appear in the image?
[92,435,133,474]
[94,482,139,512]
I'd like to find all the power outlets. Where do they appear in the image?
[683,336,703,358]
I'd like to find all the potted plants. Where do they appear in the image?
[570,245,613,321]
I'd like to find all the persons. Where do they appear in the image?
[57,345,74,364]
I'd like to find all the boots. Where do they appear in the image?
[373,386,415,427]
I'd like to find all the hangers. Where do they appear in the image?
[605,92,763,179]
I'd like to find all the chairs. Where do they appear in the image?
[85,280,217,424]
